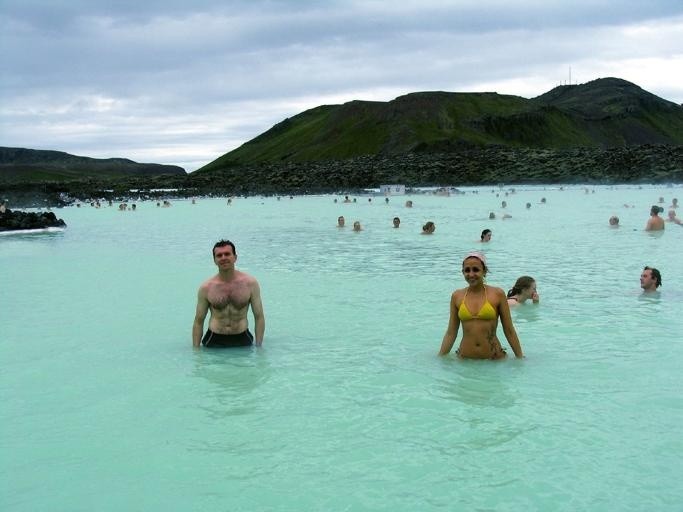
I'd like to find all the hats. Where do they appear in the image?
[463,252,487,268]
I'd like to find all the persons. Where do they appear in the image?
[0,201,6,213]
[506,276,539,306]
[192,240,265,351]
[640,266,662,294]
[438,252,523,360]
